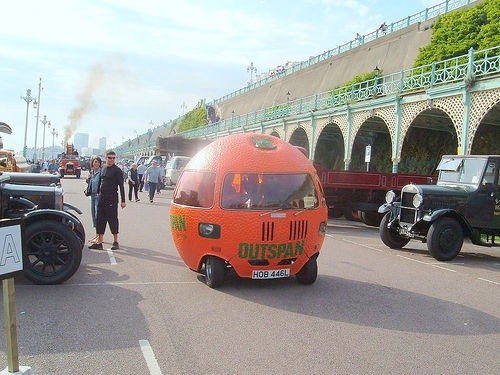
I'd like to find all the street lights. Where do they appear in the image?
[40,113,52,162]
[50,126,58,159]
[19,88,39,158]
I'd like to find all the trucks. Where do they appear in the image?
[59,144,81,180]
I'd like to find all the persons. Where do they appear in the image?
[115,157,166,193]
[83,155,103,244]
[47,159,59,174]
[128,163,141,202]
[145,159,164,203]
[87,150,128,250]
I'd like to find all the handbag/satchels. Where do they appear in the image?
[84,187,91,196]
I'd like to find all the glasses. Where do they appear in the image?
[107,157,116,159]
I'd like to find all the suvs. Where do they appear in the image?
[380,154,500,261]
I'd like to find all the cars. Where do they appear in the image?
[0,170,85,285]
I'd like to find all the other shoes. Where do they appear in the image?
[88,236,97,242]
[88,243,103,249]
[111,242,119,250]
[90,237,104,243]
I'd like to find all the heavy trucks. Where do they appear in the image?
[294,146,436,223]
